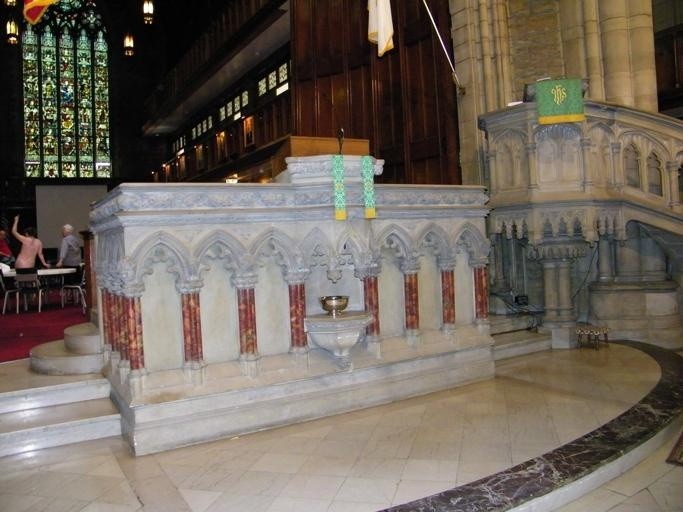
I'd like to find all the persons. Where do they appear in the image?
[56,223,82,304]
[0,229,14,269]
[11,213,51,269]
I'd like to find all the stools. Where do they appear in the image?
[577,325,609,350]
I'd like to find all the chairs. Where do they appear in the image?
[0,252,88,316]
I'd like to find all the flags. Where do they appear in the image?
[366,0,397,58]
[532,77,586,123]
[21,1,58,25]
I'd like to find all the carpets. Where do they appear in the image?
[0,289,90,367]
